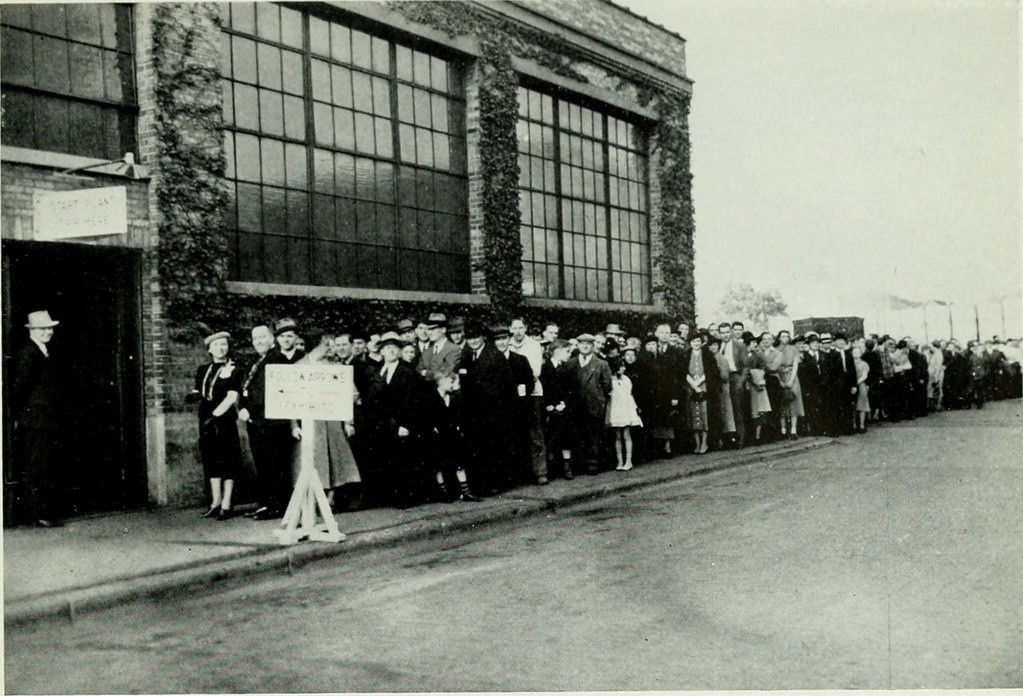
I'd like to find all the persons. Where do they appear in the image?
[236,313,1022,504]
[192,328,241,521]
[19,312,70,531]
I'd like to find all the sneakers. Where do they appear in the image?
[565,472,574,480]
[538,477,551,484]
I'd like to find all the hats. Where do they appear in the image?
[832,333,848,343]
[198,320,230,350]
[424,313,449,326]
[576,333,597,344]
[670,325,682,336]
[462,323,483,339]
[685,330,702,343]
[805,334,820,344]
[741,331,758,343]
[820,333,833,344]
[25,310,60,328]
[640,333,658,348]
[620,346,638,356]
[376,331,403,352]
[604,324,626,335]
[448,316,465,332]
[349,329,371,343]
[493,327,513,340]
[274,318,298,336]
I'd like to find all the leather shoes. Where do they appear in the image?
[243,505,283,520]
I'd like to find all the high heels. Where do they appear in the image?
[200,502,234,521]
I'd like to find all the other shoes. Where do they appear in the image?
[663,451,674,460]
[694,446,708,454]
[616,465,632,470]
[460,494,484,503]
[791,433,799,441]
[852,427,867,434]
